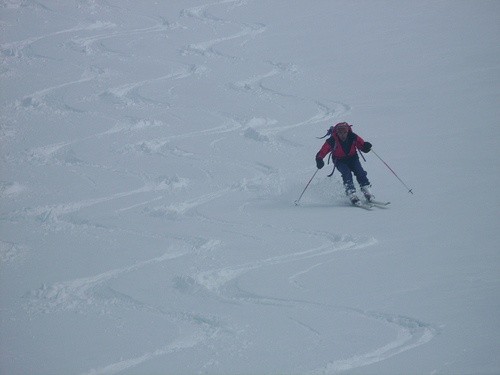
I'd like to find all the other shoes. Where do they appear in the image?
[347,191,359,203]
[361,186,375,201]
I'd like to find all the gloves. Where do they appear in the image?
[363,142,372,153]
[317,159,324,169]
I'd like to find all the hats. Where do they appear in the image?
[336,123,348,131]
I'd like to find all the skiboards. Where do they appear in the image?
[359,200,390,209]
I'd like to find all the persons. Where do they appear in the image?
[315,121,376,206]
